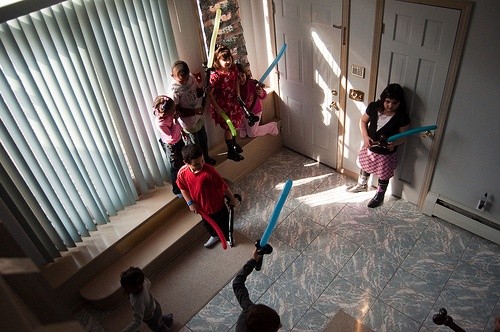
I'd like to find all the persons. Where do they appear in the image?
[170,61,217,166]
[153,96,184,199]
[120,267,173,332]
[175,145,236,246]
[207,44,245,162]
[234,63,280,138]
[233,249,282,332]
[349,83,409,208]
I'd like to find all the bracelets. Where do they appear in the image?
[187,201,193,206]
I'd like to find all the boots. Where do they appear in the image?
[367,183,388,208]
[232,136,244,154]
[225,141,244,161]
[347,174,369,193]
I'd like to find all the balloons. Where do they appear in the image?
[229,193,243,247]
[221,112,237,136]
[371,125,437,148]
[202,9,221,86]
[255,180,292,271]
[251,43,287,112]
[196,208,227,249]
